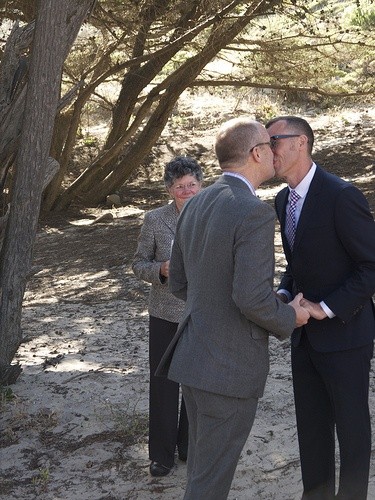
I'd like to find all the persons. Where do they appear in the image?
[267,115,375,500]
[155,117,310,500]
[132,156,203,477]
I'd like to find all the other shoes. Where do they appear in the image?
[150,460,170,476]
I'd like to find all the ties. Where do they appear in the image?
[287,190,301,257]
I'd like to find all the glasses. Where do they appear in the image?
[270,135,300,143]
[250,142,274,153]
